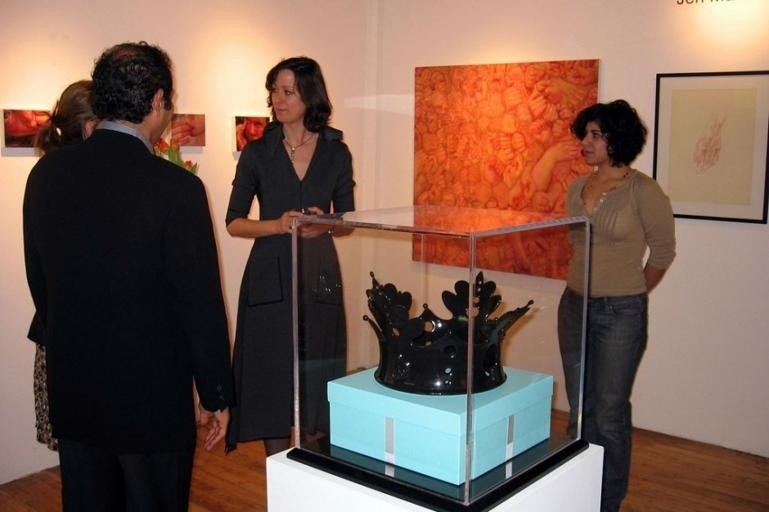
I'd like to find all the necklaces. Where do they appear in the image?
[284,132,314,162]
[582,166,632,207]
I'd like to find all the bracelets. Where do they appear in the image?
[327,224,335,235]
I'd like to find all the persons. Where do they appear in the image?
[555,97,677,512]
[24,80,97,453]
[170,113,205,146]
[235,116,270,151]
[223,55,358,458]
[24,40,234,512]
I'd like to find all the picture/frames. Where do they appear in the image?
[652,70,769,224]
